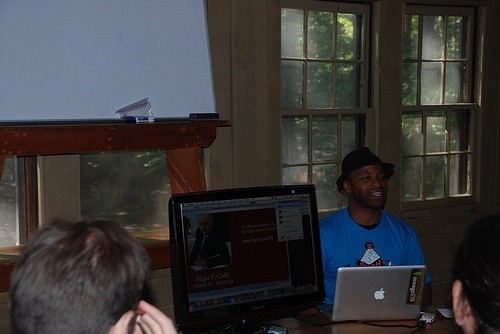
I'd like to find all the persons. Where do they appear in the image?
[10,216,176,334]
[190,214,229,269]
[313,146,431,312]
[450,214,500,334]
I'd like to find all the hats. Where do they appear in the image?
[336,147,395,194]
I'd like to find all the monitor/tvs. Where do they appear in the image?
[168,184,325,334]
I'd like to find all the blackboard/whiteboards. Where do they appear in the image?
[0,0,217,123]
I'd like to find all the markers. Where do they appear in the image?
[122,115,154,122]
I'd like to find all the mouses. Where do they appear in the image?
[435,307,454,319]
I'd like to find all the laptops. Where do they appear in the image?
[315,265,426,322]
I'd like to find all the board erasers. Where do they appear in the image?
[188,113,219,121]
